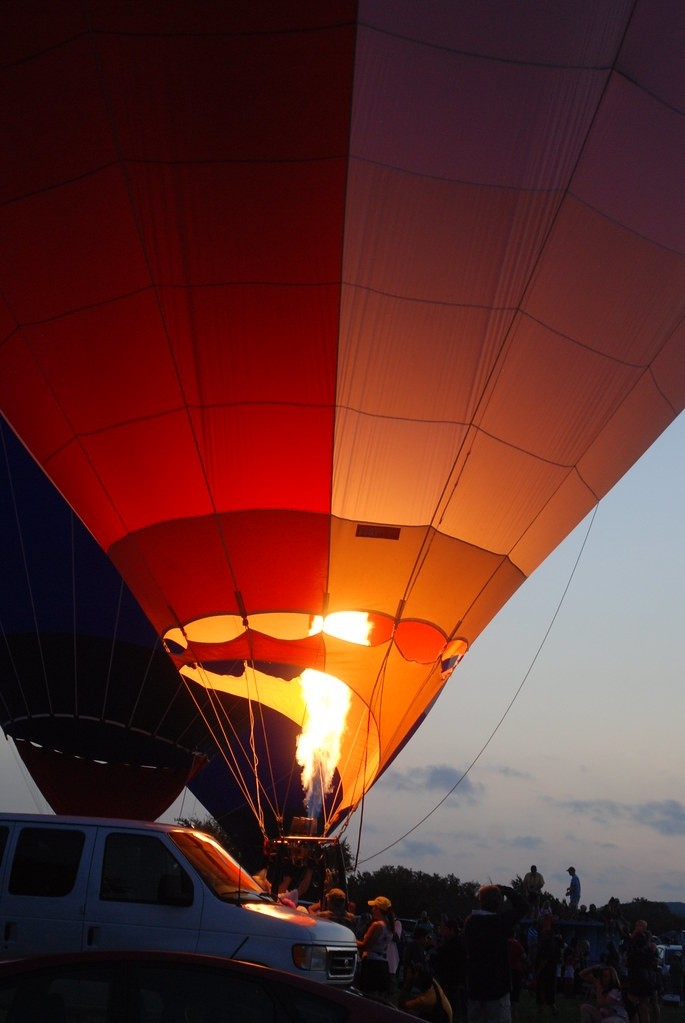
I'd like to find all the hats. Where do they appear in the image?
[566,867,576,871]
[478,885,506,904]
[325,888,345,899]
[367,896,391,909]
[422,911,427,918]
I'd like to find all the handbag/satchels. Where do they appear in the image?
[416,1004,449,1023]
[387,942,400,975]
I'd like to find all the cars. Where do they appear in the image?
[0,949,432,1023]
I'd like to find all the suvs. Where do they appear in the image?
[656,943,682,979]
[0,812,358,987]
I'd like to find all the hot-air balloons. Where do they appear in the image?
[184,686,445,885]
[0,420,228,823]
[0,0,685,893]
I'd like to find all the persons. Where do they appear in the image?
[523,865,545,896]
[566,866,581,907]
[252,869,685,1023]
